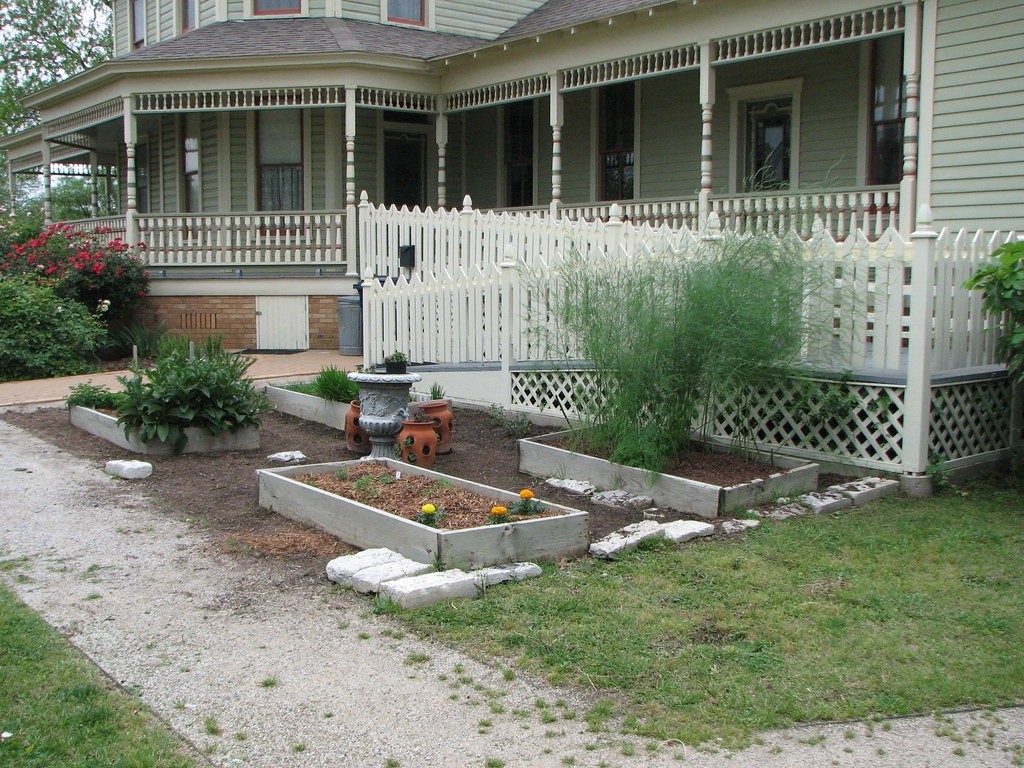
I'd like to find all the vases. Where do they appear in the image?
[347,372,422,461]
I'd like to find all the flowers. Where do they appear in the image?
[419,489,544,528]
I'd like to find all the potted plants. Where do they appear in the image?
[393,409,438,469]
[418,402,453,454]
[517,156,871,518]
[385,352,407,374]
[67,341,272,455]
[264,363,455,434]
[345,395,372,454]
